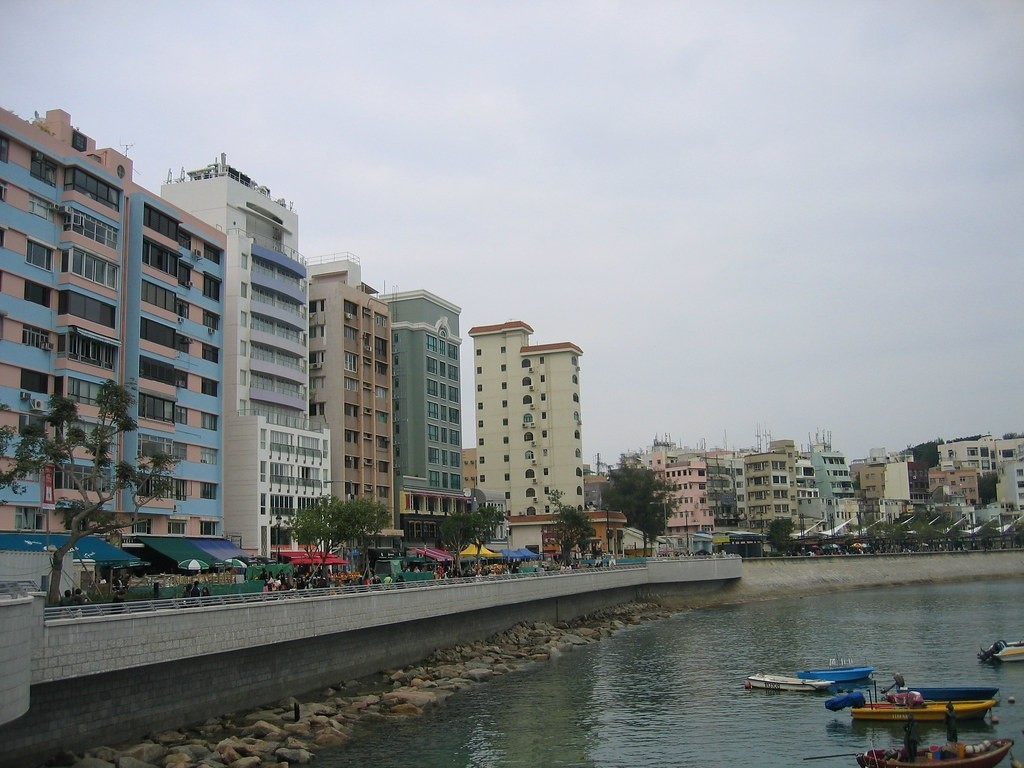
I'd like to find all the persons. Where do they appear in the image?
[196,567,237,576]
[903,713,922,762]
[110,591,125,614]
[785,545,1024,553]
[260,567,406,597]
[556,551,607,568]
[182,581,211,608]
[95,575,136,590]
[59,588,84,605]
[675,551,693,557]
[944,700,956,742]
[407,561,519,580]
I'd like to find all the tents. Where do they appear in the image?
[462,537,503,565]
[500,547,539,564]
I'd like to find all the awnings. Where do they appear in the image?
[279,551,347,565]
[396,547,455,561]
[1,533,151,567]
[136,536,249,568]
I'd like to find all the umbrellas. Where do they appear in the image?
[178,558,209,570]
[224,558,247,568]
[850,543,867,550]
[822,543,838,550]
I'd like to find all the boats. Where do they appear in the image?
[829,691,997,723]
[976,640,1024,662]
[895,683,1000,702]
[855,738,1014,768]
[747,672,836,692]
[796,666,880,683]
